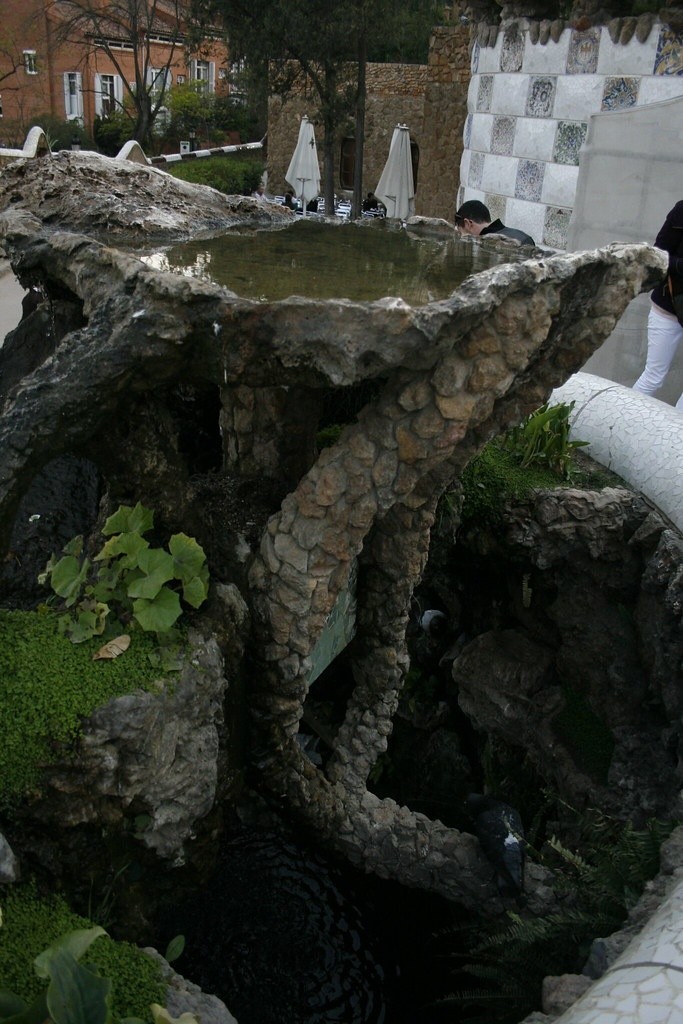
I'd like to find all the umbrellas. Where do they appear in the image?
[285,116,321,216]
[374,124,416,224]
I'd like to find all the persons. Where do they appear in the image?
[307,200,318,212]
[363,193,377,212]
[255,186,269,202]
[631,199,683,408]
[282,194,298,210]
[456,200,536,247]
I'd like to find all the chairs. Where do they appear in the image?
[260,194,385,222]
